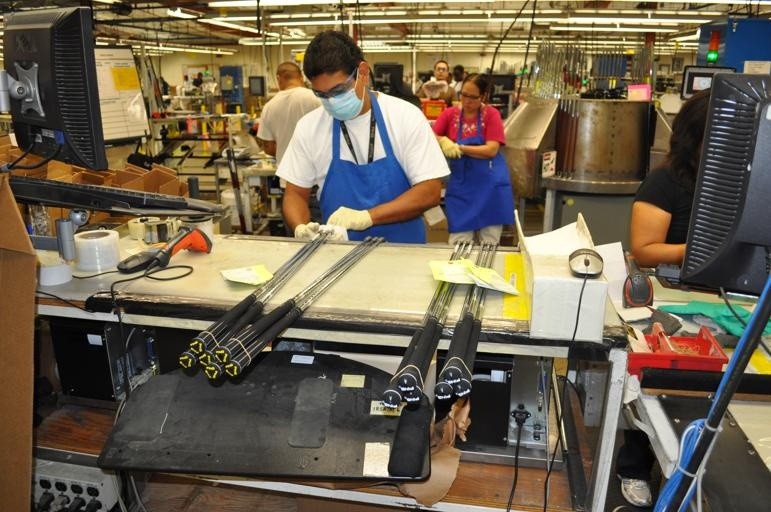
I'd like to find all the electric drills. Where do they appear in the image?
[146,223,212,271]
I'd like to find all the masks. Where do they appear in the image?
[321,67,365,121]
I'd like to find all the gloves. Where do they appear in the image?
[439,136,463,159]
[326,207,373,231]
[294,223,342,241]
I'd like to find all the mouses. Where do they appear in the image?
[117,253,153,274]
[569,249,603,278]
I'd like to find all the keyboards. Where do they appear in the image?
[654,264,682,278]
[9,175,188,212]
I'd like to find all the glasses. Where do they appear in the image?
[312,67,357,98]
[460,91,482,100]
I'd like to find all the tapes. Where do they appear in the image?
[74,229,120,271]
[39,264,73,286]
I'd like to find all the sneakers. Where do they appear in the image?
[616,473,652,507]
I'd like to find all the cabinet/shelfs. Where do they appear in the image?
[212,153,264,209]
[240,161,322,237]
[145,112,249,196]
[32,223,630,512]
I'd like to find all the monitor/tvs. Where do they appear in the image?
[3,6,108,171]
[248,76,264,97]
[374,64,403,96]
[484,74,514,103]
[680,65,736,100]
[93,44,152,146]
[679,73,771,296]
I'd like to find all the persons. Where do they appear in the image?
[275,29,453,245]
[256,62,323,237]
[158,72,204,96]
[430,73,516,245]
[414,60,468,108]
[629,87,712,268]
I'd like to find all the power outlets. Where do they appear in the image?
[33,459,122,512]
